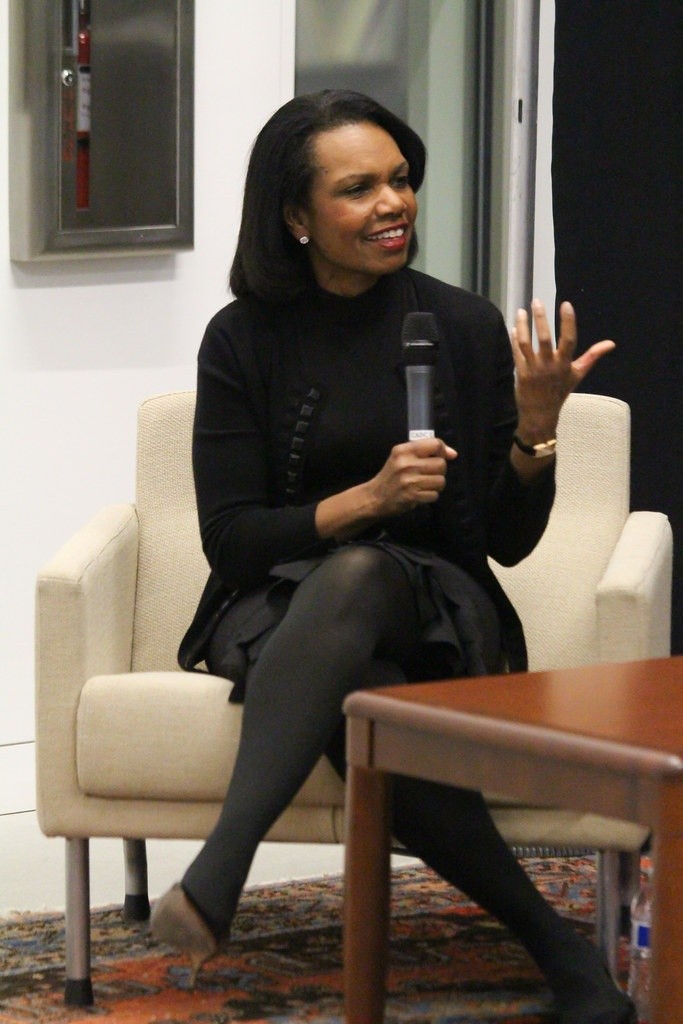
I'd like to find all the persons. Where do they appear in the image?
[146,90,637,1024]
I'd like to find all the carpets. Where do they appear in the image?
[0,853,658,1023]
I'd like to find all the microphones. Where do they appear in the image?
[400,310,439,443]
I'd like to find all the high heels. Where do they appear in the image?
[151,885,220,988]
[565,955,637,1023]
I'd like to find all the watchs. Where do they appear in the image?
[513,431,557,457]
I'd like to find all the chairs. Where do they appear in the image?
[33,392,673,1004]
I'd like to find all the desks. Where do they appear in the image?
[343,654,682,1024]
